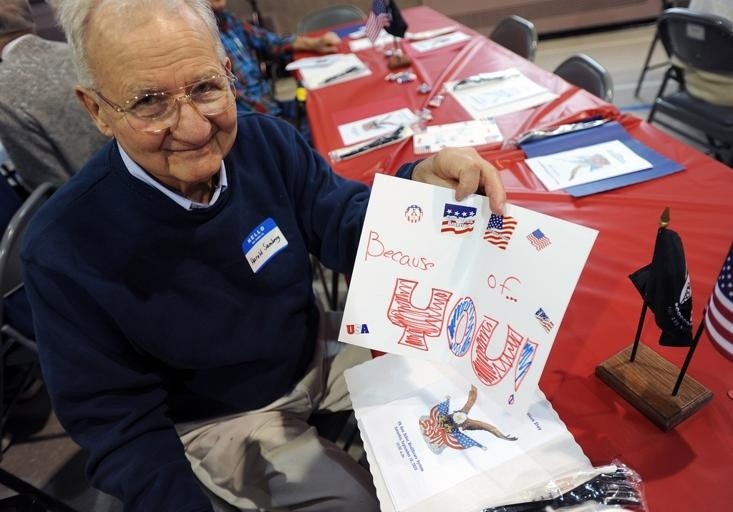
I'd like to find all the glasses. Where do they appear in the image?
[92,65,238,135]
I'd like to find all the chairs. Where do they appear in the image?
[489,15,536,63]
[647,8,733,167]
[552,53,613,106]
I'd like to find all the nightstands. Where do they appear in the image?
[297,8,366,35]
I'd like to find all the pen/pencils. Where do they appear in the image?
[319,66,357,86]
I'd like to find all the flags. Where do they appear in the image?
[533,308,556,334]
[482,212,518,251]
[628,227,733,362]
[524,228,552,250]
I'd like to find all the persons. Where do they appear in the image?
[655,0,733,107]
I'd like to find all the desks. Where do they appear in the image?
[293,7,733,508]
[290,7,733,511]
